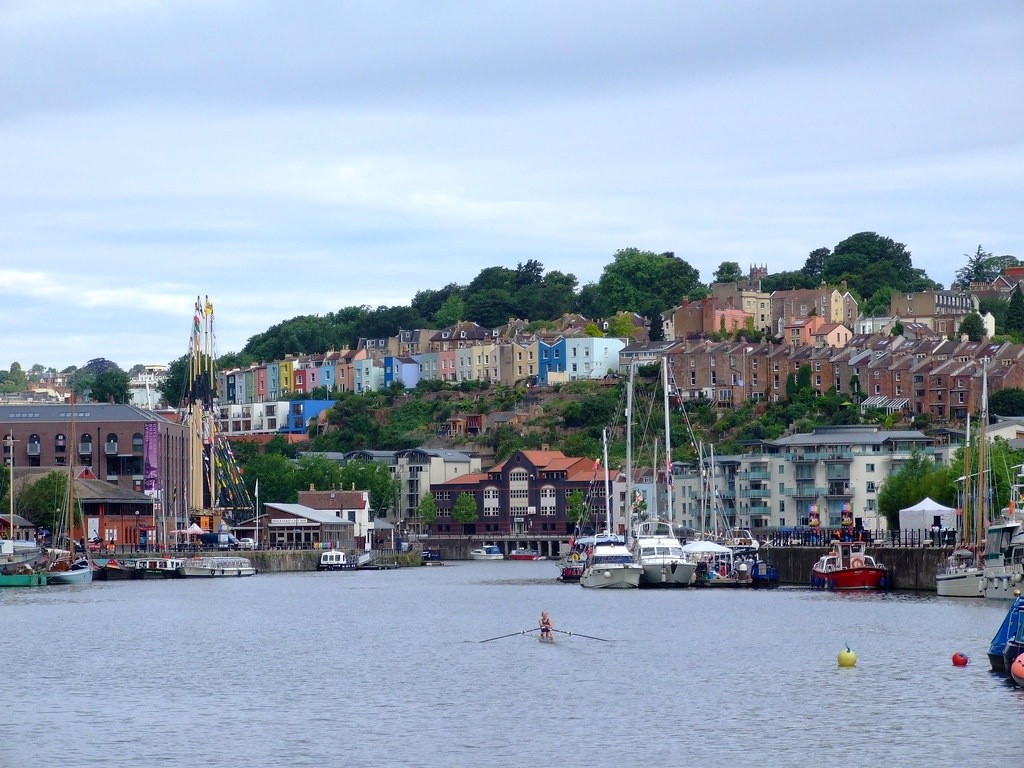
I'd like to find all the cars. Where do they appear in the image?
[238,539,258,550]
[43,536,82,552]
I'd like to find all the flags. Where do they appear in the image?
[591,459,600,470]
[203,405,244,500]
[636,491,643,506]
[188,303,205,353]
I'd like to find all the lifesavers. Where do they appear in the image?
[852,557,865,568]
[572,554,578,561]
[238,570,241,576]
[255,569,258,575]
[222,569,225,574]
[211,569,215,574]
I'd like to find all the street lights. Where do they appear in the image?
[396,520,403,537]
[56,506,60,537]
[135,511,139,552]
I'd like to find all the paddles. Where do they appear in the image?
[479,627,545,643]
[550,629,606,642]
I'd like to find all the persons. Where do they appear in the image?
[110,538,114,544]
[540,611,553,636]
[7,554,13,562]
[140,537,146,553]
[810,513,817,522]
[930,524,948,547]
[842,513,850,522]
[80,536,85,554]
[834,523,864,541]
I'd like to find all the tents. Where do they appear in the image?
[171,524,209,544]
[899,497,957,546]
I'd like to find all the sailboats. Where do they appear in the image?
[0,385,183,586]
[937,355,1023,600]
[375,450,443,564]
[555,354,782,590]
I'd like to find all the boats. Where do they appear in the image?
[133,558,182,579]
[177,557,257,576]
[810,541,888,590]
[98,559,140,581]
[510,548,540,562]
[471,545,503,561]
[318,551,357,571]
